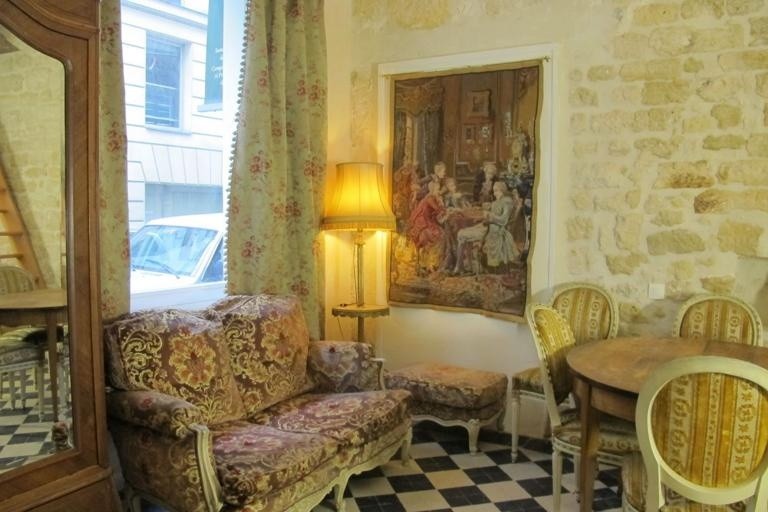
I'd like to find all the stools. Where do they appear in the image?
[383,363,507,456]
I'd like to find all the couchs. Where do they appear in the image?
[102,293,413,512]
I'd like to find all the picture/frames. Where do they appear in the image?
[385,59,544,325]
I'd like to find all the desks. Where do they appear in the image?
[564,336,767,512]
[0,288,67,420]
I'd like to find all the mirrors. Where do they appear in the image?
[0,1,107,511]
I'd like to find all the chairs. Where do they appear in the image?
[525,302,643,512]
[670,293,764,347]
[510,283,619,464]
[0,265,71,422]
[619,355,767,512]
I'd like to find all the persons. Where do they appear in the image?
[408,161,520,276]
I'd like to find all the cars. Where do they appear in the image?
[131,213,227,293]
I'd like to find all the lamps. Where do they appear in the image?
[318,161,396,342]
